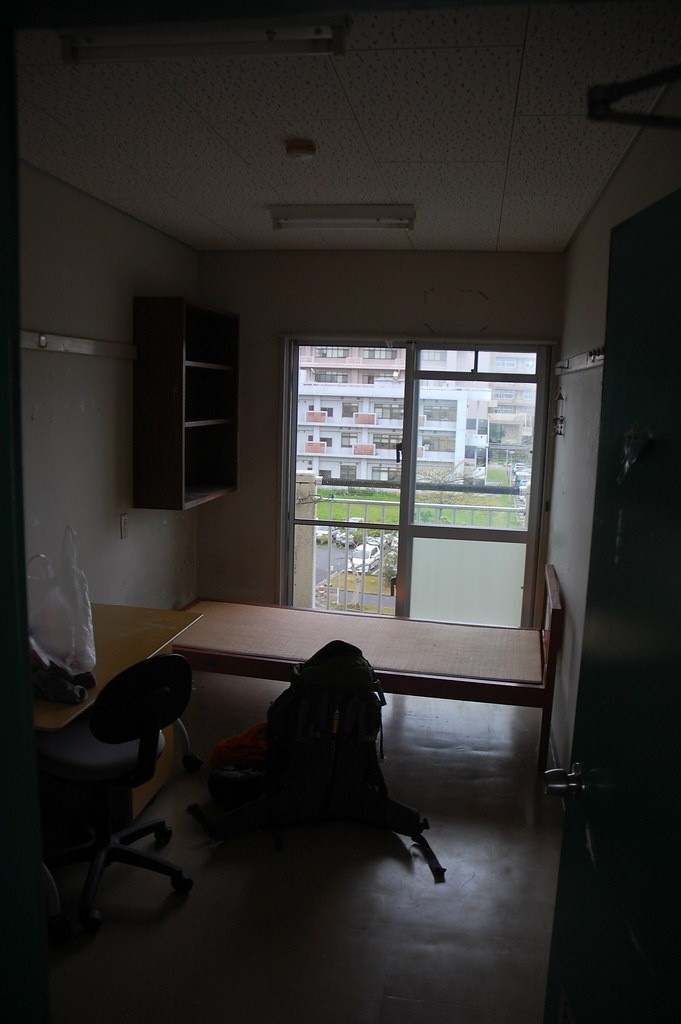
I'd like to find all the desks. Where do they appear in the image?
[36,602,207,942]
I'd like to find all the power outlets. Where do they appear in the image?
[120,513,128,539]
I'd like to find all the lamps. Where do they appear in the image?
[59,8,353,67]
[268,203,417,232]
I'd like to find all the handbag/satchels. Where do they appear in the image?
[27,525,97,676]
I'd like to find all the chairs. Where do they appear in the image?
[173,564,564,774]
[36,652,195,933]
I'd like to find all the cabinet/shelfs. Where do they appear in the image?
[133,295,241,510]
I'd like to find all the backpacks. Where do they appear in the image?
[264,641,386,808]
